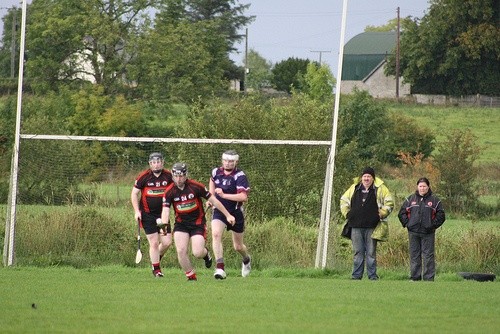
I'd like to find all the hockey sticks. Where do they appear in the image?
[135,217,143,264]
[204,205,211,215]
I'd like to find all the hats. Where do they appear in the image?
[362,166,375,180]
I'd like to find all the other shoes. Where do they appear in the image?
[153,271,164,278]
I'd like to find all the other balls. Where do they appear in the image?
[32,303,35,307]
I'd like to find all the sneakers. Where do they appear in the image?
[242,254,251,277]
[187,278,197,282]
[214,268,226,280]
[203,251,212,268]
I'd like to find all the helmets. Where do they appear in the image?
[149,153,163,172]
[221,150,239,161]
[171,163,187,176]
